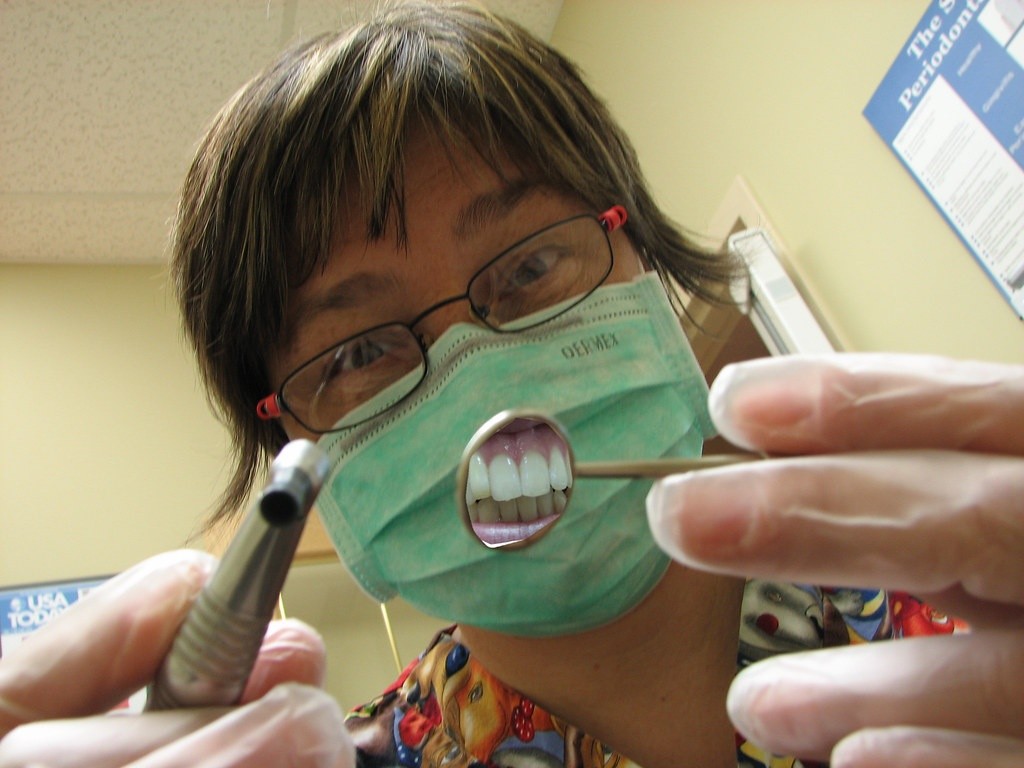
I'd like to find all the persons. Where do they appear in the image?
[0,0,1023,768]
[463,418,571,544]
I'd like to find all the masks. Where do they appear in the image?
[309,272,719,637]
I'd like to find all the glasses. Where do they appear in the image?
[258,204,629,435]
